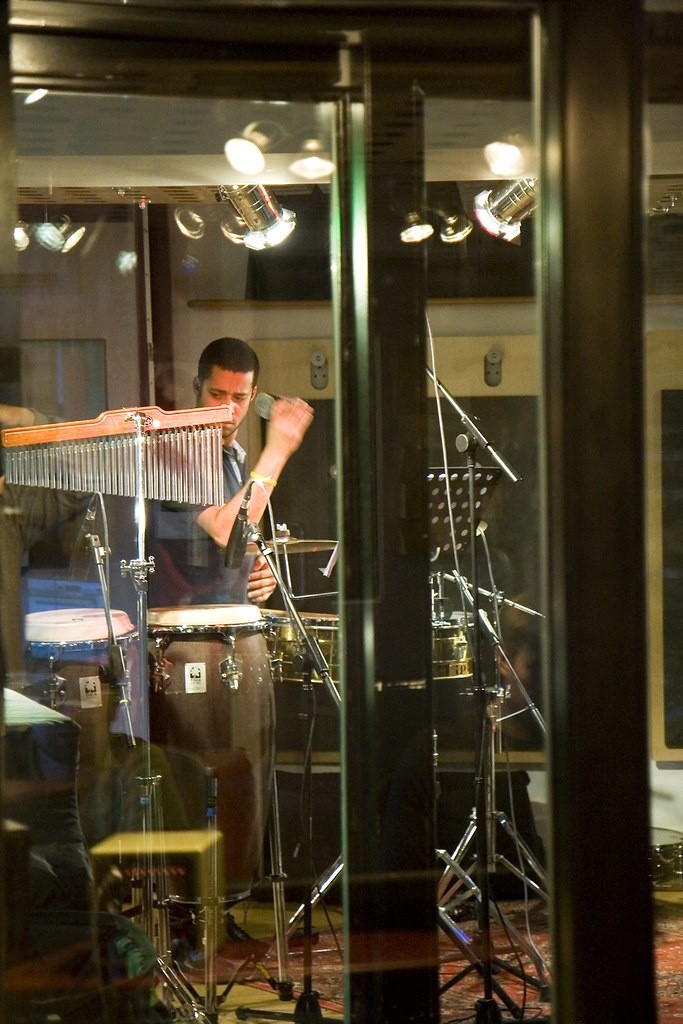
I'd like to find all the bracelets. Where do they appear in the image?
[250,471,277,487]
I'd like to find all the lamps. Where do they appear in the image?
[223,180,296,249]
[401,177,540,244]
[55,218,86,253]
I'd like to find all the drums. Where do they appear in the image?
[20,606,142,845]
[431,608,478,681]
[143,604,278,907]
[260,609,342,685]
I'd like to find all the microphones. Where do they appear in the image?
[69,497,98,582]
[224,486,252,569]
[255,393,275,420]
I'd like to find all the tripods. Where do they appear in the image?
[234,364,557,1024]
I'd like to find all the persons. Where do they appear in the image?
[145,336,315,951]
[0,403,112,972]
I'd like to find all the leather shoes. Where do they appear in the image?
[221,912,269,955]
[170,935,230,975]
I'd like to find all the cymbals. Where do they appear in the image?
[245,536,339,556]
